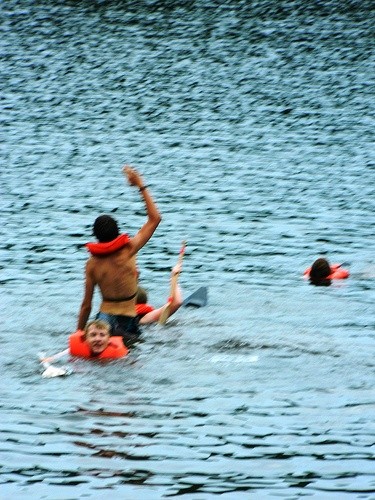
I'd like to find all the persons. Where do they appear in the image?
[76,165,162,348]
[135,239,188,326]
[39,318,128,364]
[309,258,331,279]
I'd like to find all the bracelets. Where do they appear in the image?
[139,183,151,192]
